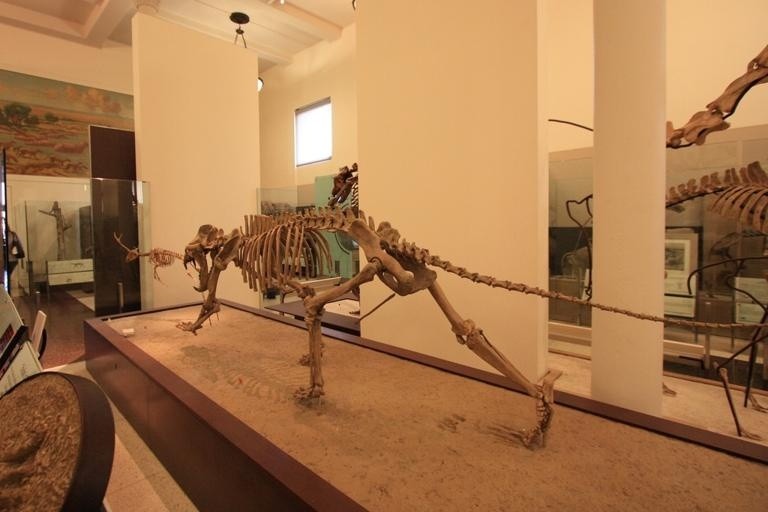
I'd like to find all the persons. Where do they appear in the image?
[4,224,26,290]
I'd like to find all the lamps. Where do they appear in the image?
[257,77,264,91]
